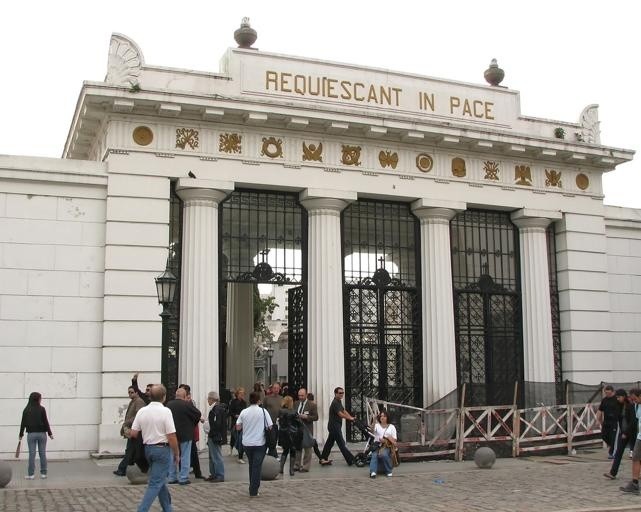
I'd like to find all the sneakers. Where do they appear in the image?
[40,475,48,479]
[602,471,617,480]
[318,454,359,466]
[237,459,247,464]
[24,475,35,480]
[369,471,394,478]
[619,480,641,495]
[628,450,634,458]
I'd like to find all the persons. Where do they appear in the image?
[370,410,397,477]
[597,386,619,458]
[113,371,332,511]
[603,389,637,479]
[19,392,53,479]
[319,387,356,465]
[619,388,641,492]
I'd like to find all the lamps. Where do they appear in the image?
[155,258,177,316]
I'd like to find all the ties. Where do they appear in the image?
[299,402,304,414]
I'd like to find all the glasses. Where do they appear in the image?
[338,392,344,394]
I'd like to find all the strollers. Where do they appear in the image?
[349,418,401,468]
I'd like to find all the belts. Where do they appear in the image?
[154,441,168,447]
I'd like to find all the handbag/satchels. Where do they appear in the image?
[391,445,401,467]
[15,441,22,458]
[264,424,279,449]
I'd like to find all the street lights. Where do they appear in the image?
[153,259,178,401]
[266,342,275,385]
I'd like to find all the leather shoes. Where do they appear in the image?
[168,473,224,486]
[280,466,310,476]
